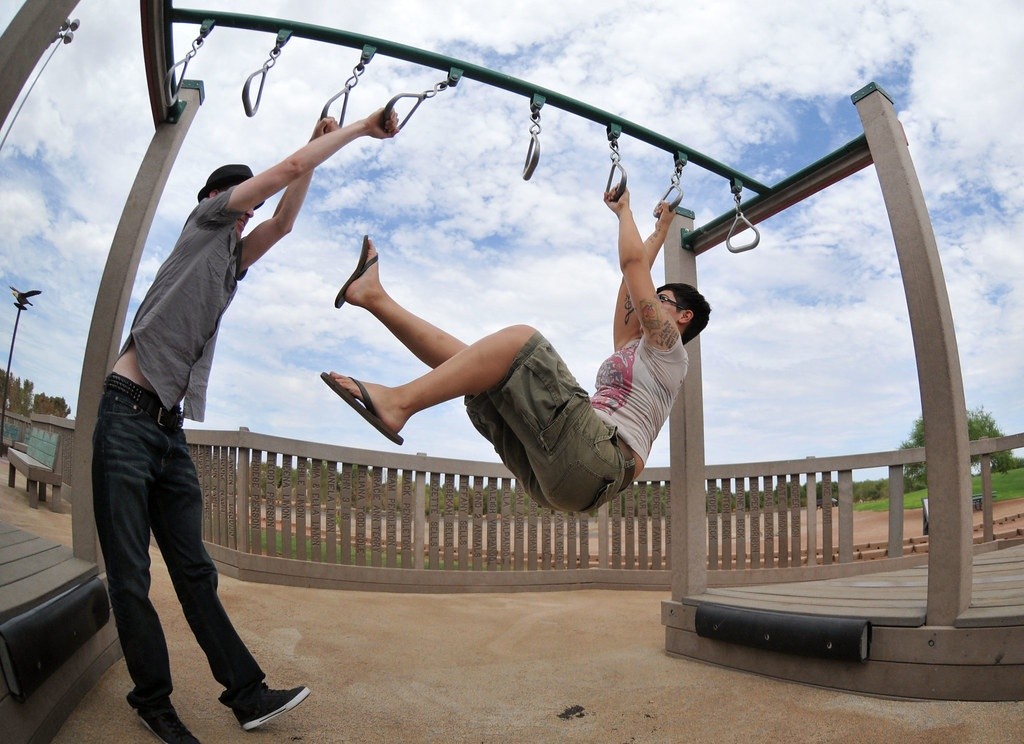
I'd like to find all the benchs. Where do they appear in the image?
[1,423,64,514]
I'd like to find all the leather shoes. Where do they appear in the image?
[103,374,184,429]
[617,436,635,494]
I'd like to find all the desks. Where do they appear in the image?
[972,490,998,510]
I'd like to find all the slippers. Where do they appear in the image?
[334,235,378,309]
[320,372,404,446]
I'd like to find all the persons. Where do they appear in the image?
[323,181,711,515]
[91,103,400,743]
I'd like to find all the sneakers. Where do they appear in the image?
[231,682,310,730]
[137,709,199,744]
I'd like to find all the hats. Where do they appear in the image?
[197,164,265,211]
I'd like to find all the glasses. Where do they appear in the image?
[658,294,685,309]
